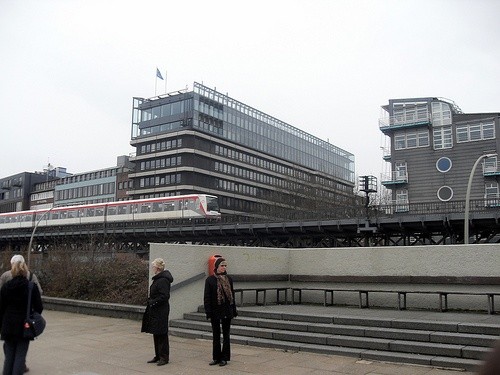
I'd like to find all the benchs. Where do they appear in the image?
[234,288,500,316]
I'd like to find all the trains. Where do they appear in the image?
[0,192,223,242]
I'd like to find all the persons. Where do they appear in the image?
[0,254,44,375]
[140,257,174,366]
[203,258,238,366]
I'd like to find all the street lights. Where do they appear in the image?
[464,153,498,244]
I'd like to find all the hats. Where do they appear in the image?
[151,257,166,269]
[213,257,226,270]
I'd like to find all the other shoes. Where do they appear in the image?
[209,359,227,367]
[147,356,169,366]
[24,365,30,372]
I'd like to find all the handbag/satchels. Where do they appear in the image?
[23,311,46,341]
[223,304,239,321]
